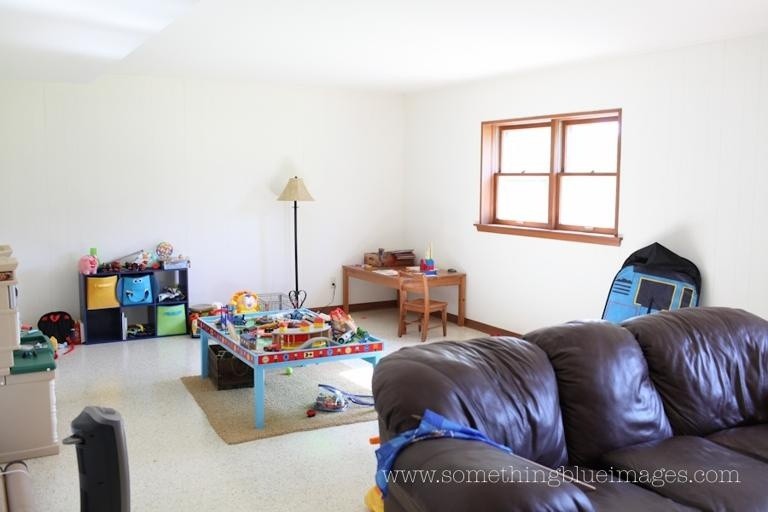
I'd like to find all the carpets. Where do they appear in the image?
[180,358,378,445]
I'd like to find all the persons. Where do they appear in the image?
[17,239,188,362]
[186,290,386,429]
[419,258,437,272]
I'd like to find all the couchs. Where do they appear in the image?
[370,305,767,512]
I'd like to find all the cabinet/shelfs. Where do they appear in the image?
[78,262,190,345]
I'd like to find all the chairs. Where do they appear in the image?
[397,270,449,343]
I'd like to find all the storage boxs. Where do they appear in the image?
[0,328,61,464]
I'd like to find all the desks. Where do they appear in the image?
[196,308,386,430]
[342,264,466,326]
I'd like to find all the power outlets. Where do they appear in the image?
[328,277,335,289]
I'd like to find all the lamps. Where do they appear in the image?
[276,175,315,309]
